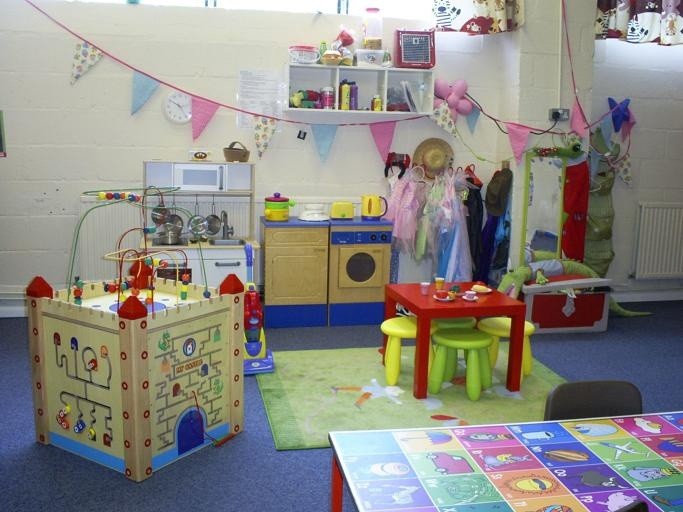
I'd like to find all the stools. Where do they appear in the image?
[475,318,539,386]
[429,315,476,383]
[378,317,434,385]
[426,330,494,401]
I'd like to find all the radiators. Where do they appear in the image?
[632,200,683,281]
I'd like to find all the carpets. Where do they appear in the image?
[258,342,569,448]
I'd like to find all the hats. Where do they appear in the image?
[411,137,455,182]
[484,168,512,217]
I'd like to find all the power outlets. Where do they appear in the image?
[548,108,571,121]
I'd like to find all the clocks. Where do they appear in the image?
[161,94,196,125]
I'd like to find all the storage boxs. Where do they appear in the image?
[510,264,612,333]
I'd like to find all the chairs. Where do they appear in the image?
[539,379,643,420]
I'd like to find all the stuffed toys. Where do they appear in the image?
[434,79,472,125]
[608,97,631,133]
[494,259,654,316]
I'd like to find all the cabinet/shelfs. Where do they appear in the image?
[137,240,261,290]
[257,216,331,329]
[264,56,435,130]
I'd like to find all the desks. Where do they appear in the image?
[383,280,526,392]
[311,411,683,512]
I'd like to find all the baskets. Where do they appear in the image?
[223,141,250,163]
